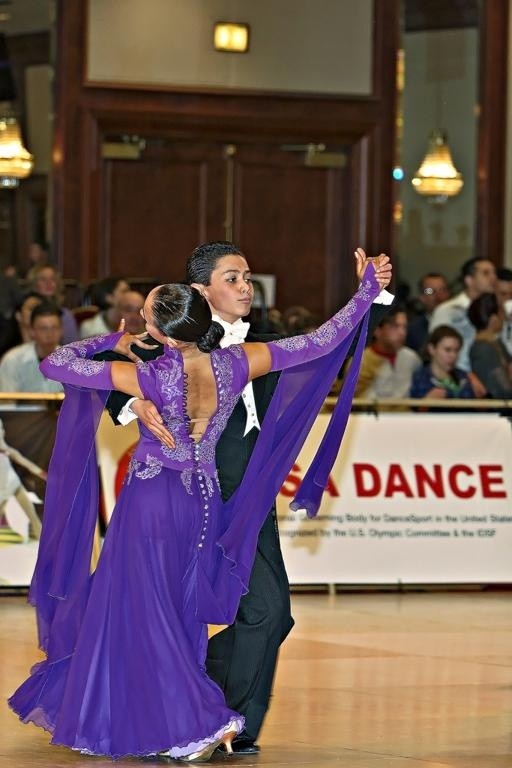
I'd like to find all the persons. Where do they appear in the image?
[0,242,512,400]
[88,238,394,756]
[39,245,382,763]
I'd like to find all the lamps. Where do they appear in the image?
[211,19,250,52]
[409,94,463,205]
[1,99,33,189]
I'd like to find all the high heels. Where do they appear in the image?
[177,719,239,762]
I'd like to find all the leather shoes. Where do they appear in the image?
[215,737,260,755]
